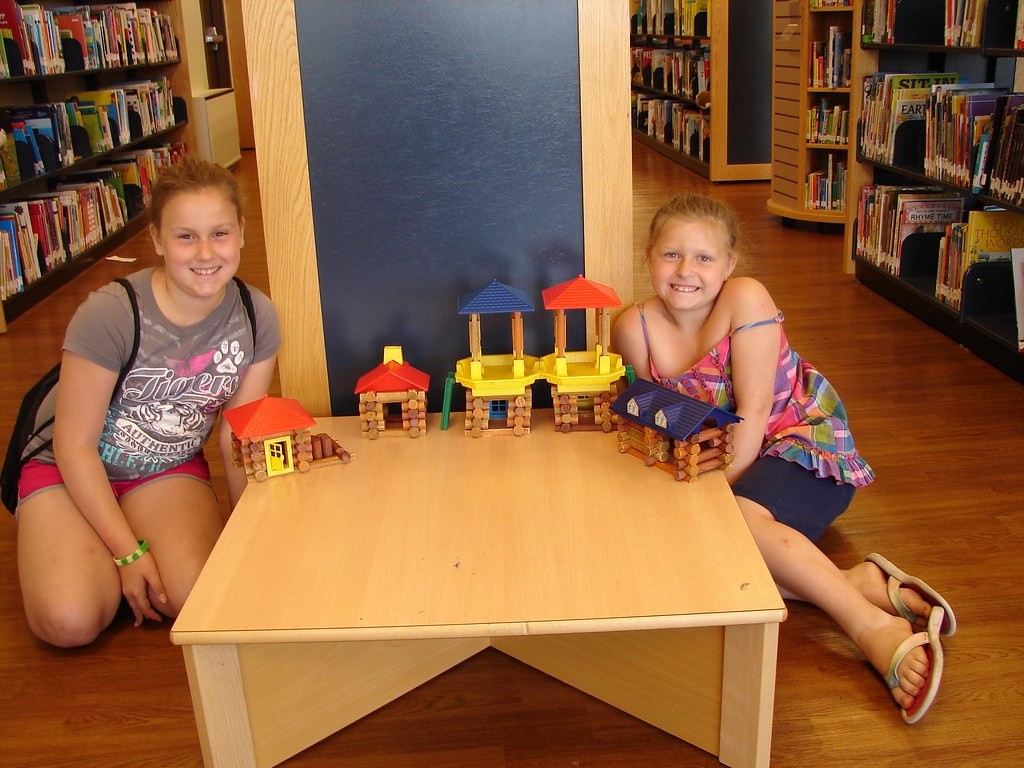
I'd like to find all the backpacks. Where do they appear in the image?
[0,276,256,515]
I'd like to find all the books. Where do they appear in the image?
[806,98,848,145]
[0,142,186,302]
[630,45,711,101]
[630,0,711,38]
[805,154,847,211]
[0,76,176,192]
[0,0,179,79]
[857,184,1024,350]
[861,0,1024,50]
[857,72,1024,206]
[809,0,855,8]
[631,90,710,162]
[812,25,851,88]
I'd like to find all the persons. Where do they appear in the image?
[15,159,284,649]
[615,195,956,725]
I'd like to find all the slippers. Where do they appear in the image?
[882,606,945,722]
[864,551,956,637]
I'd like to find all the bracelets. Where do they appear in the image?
[113,539,148,566]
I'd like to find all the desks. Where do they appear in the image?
[169,406,788,767]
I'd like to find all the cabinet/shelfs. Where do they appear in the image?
[630,0,1024,383]
[0,0,244,328]
